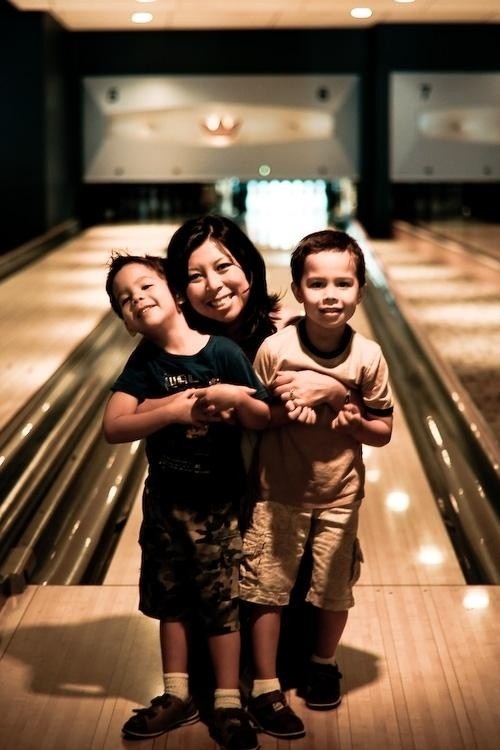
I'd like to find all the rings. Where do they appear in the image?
[288,386,298,401]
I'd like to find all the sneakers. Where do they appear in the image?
[208,707,262,750]
[122,688,200,738]
[304,660,343,709]
[247,688,306,739]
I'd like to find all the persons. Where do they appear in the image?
[250,228,395,739]
[103,255,274,748]
[164,213,364,706]
